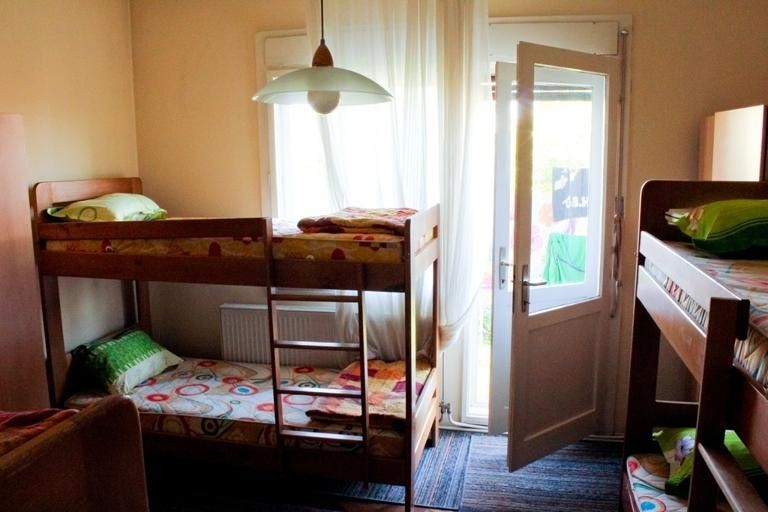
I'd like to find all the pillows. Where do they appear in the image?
[48,191,167,225]
[660,194,765,257]
[75,325,184,397]
[647,426,759,495]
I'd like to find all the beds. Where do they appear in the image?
[28,174,446,509]
[0,394,152,510]
[617,178,768,512]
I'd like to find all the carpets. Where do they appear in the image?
[330,428,471,511]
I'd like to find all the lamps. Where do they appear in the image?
[250,3,392,117]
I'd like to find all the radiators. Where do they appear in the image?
[218,304,339,369]
